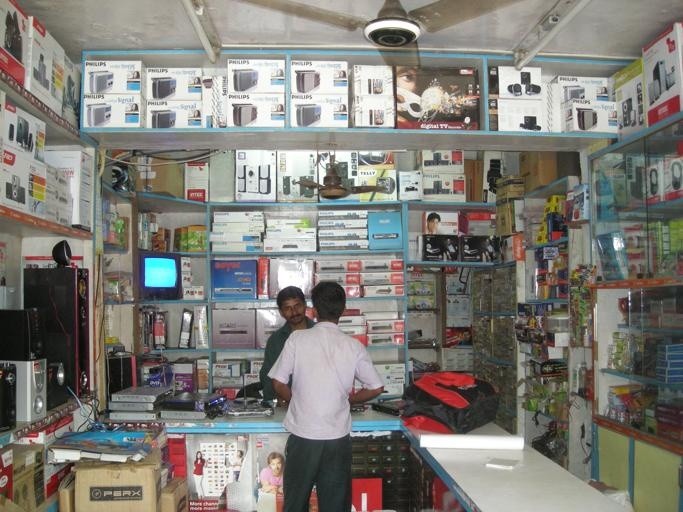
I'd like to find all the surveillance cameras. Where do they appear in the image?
[541,16,560,31]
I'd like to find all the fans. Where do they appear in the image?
[231,0,527,69]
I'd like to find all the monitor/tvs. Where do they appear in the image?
[138,252,183,300]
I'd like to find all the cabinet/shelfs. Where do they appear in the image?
[101,179,406,415]
[475,260,526,436]
[405,200,500,268]
[442,272,474,371]
[160,403,407,512]
[78,48,638,155]
[0,67,111,512]
[400,420,633,512]
[521,175,593,482]
[406,270,442,379]
[585,112,682,511]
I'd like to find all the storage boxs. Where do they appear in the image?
[3,101,46,162]
[349,96,396,127]
[269,257,316,300]
[0,446,13,501]
[290,95,349,128]
[27,15,65,122]
[148,101,203,129]
[169,87,178,88]
[488,98,547,132]
[641,22,683,127]
[183,161,208,202]
[291,59,348,95]
[224,272,227,274]
[496,175,526,206]
[226,94,285,127]
[255,309,289,350]
[519,150,558,194]
[7,443,45,512]
[227,59,285,94]
[146,67,203,101]
[83,60,147,99]
[488,65,542,99]
[358,149,398,203]
[394,65,481,130]
[608,57,648,141]
[367,211,404,250]
[35,144,95,231]
[414,149,465,174]
[19,412,75,501]
[211,260,257,299]
[276,150,319,202]
[0,144,45,218]
[318,150,360,204]
[0,0,29,89]
[561,98,618,133]
[541,83,561,132]
[73,465,158,512]
[253,80,261,84]
[62,53,80,127]
[83,94,147,131]
[494,201,516,237]
[234,148,278,202]
[499,232,525,265]
[549,75,608,104]
[211,309,257,349]
[399,170,422,201]
[348,64,394,96]
[422,173,466,202]
[129,154,186,198]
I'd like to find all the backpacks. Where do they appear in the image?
[401,371,500,434]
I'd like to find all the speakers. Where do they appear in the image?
[52,239,72,267]
[47,360,67,411]
[0,364,16,433]
[23,268,90,411]
[1,309,48,361]
[0,359,47,422]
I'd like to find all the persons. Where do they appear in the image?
[230,450,244,481]
[396,66,424,122]
[259,286,316,402]
[192,451,208,500]
[267,281,384,512]
[427,212,441,234]
[260,452,285,493]
